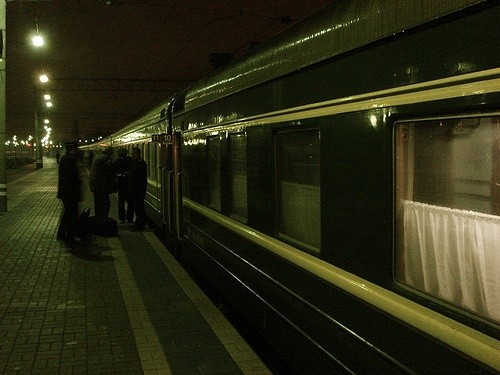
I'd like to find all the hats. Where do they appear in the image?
[64,139,79,150]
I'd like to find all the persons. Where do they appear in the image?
[86,150,94,169]
[55,139,84,241]
[89,146,115,217]
[55,151,60,163]
[127,147,148,230]
[113,148,132,224]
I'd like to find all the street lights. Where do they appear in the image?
[29,16,45,169]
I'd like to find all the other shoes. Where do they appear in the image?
[128,219,134,223]
[57,234,67,239]
[66,239,79,247]
[119,219,125,224]
[133,224,145,230]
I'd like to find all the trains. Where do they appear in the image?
[56,0,500,375]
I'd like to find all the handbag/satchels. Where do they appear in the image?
[89,215,118,236]
[78,207,92,237]
